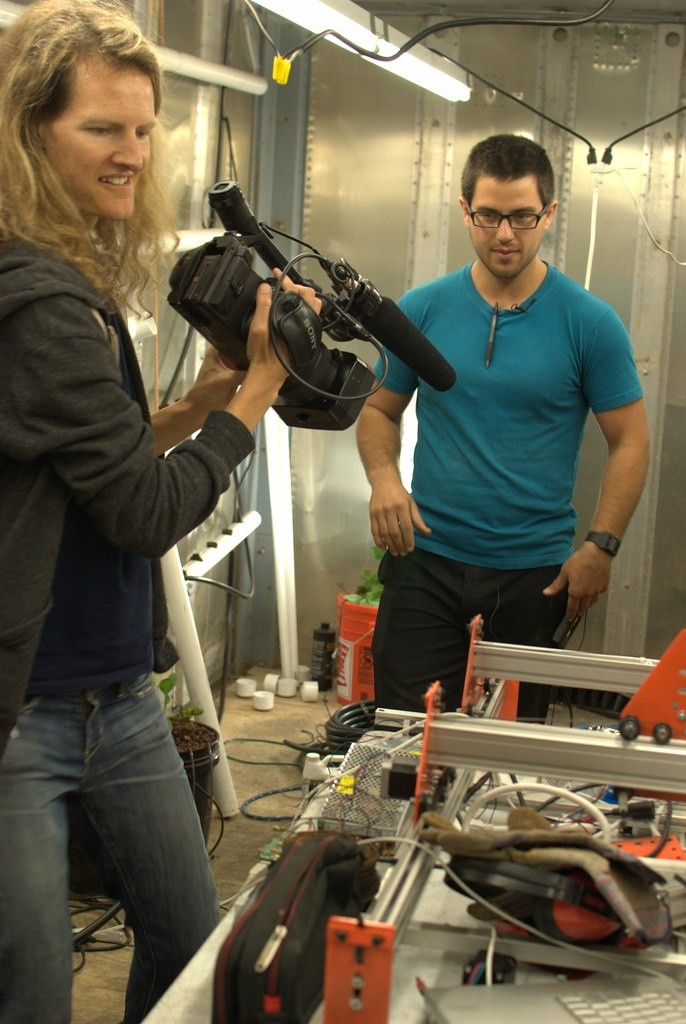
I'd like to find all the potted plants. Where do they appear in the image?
[152,672,220,849]
[331,546,386,706]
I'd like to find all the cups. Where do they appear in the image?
[235,664,319,710]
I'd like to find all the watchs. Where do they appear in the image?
[584,531,620,557]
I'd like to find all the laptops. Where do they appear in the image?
[429,971,686,1024]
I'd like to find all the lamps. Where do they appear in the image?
[251,0,473,102]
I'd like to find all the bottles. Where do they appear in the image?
[312,622,336,691]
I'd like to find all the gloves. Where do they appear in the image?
[418,808,672,945]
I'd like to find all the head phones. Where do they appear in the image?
[444,855,625,966]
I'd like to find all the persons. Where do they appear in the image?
[356,135,651,800]
[0,1,325,1024]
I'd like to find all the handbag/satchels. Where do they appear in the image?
[214,829,382,1024]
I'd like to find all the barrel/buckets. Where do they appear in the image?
[302,752,344,798]
[336,592,379,706]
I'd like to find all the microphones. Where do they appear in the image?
[363,297,457,393]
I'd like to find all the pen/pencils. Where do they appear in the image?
[485,302,499,368]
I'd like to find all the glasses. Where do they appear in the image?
[465,199,549,229]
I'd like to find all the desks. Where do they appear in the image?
[141,770,686,1024]
